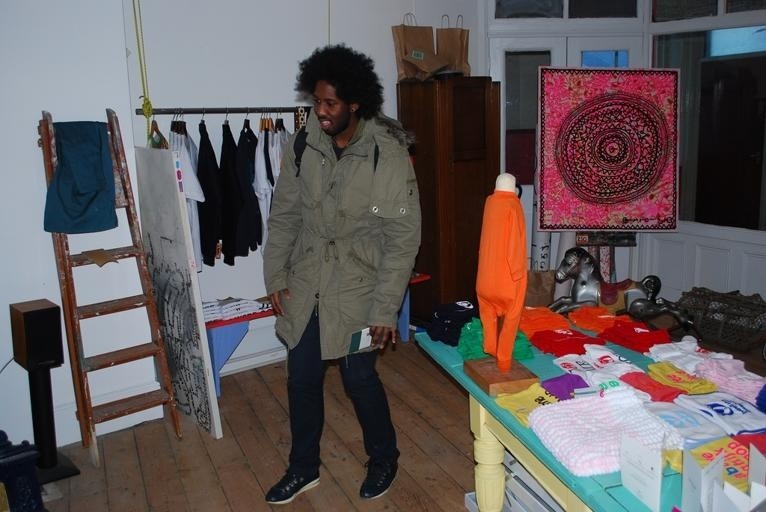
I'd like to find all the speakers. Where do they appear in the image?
[10,299,64,373]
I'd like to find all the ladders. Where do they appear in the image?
[36,110,183,468]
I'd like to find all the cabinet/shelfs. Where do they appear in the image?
[388,70,518,344]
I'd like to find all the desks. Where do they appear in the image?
[408,301,766,511]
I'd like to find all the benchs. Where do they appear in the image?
[197,265,440,401]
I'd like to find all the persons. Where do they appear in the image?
[263,43,423,507]
[475,171,528,373]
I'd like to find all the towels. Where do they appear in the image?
[40,120,120,234]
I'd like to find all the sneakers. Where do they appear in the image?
[359,447,402,499]
[265,465,321,505]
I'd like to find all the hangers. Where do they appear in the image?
[138,104,318,132]
